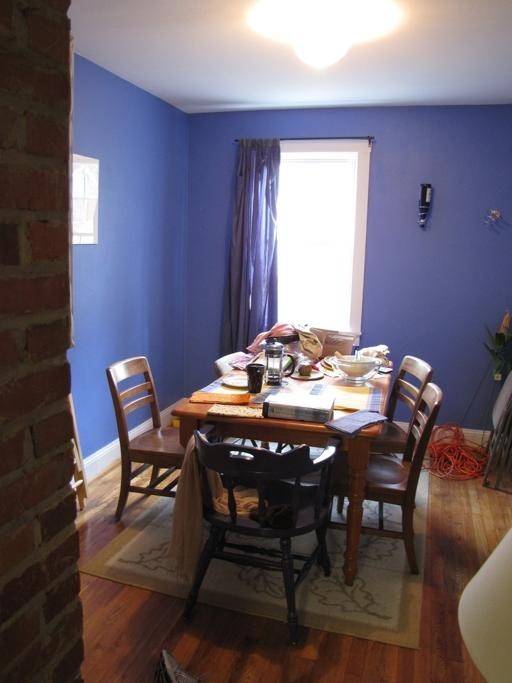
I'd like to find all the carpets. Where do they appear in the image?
[78,438,432,650]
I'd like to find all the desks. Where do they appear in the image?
[171,359,394,587]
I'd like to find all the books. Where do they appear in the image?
[263,393,336,422]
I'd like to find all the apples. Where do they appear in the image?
[298,362,312,376]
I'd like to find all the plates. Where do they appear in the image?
[224,375,253,389]
[290,371,326,381]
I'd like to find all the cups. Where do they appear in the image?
[266,338,295,385]
[247,364,264,393]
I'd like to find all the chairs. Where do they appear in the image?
[214,351,258,448]
[312,328,356,357]
[106,356,224,521]
[337,354,434,514]
[173,424,340,649]
[315,382,444,575]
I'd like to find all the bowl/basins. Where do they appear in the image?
[329,355,382,386]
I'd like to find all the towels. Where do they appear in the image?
[325,408,390,439]
[189,390,251,406]
